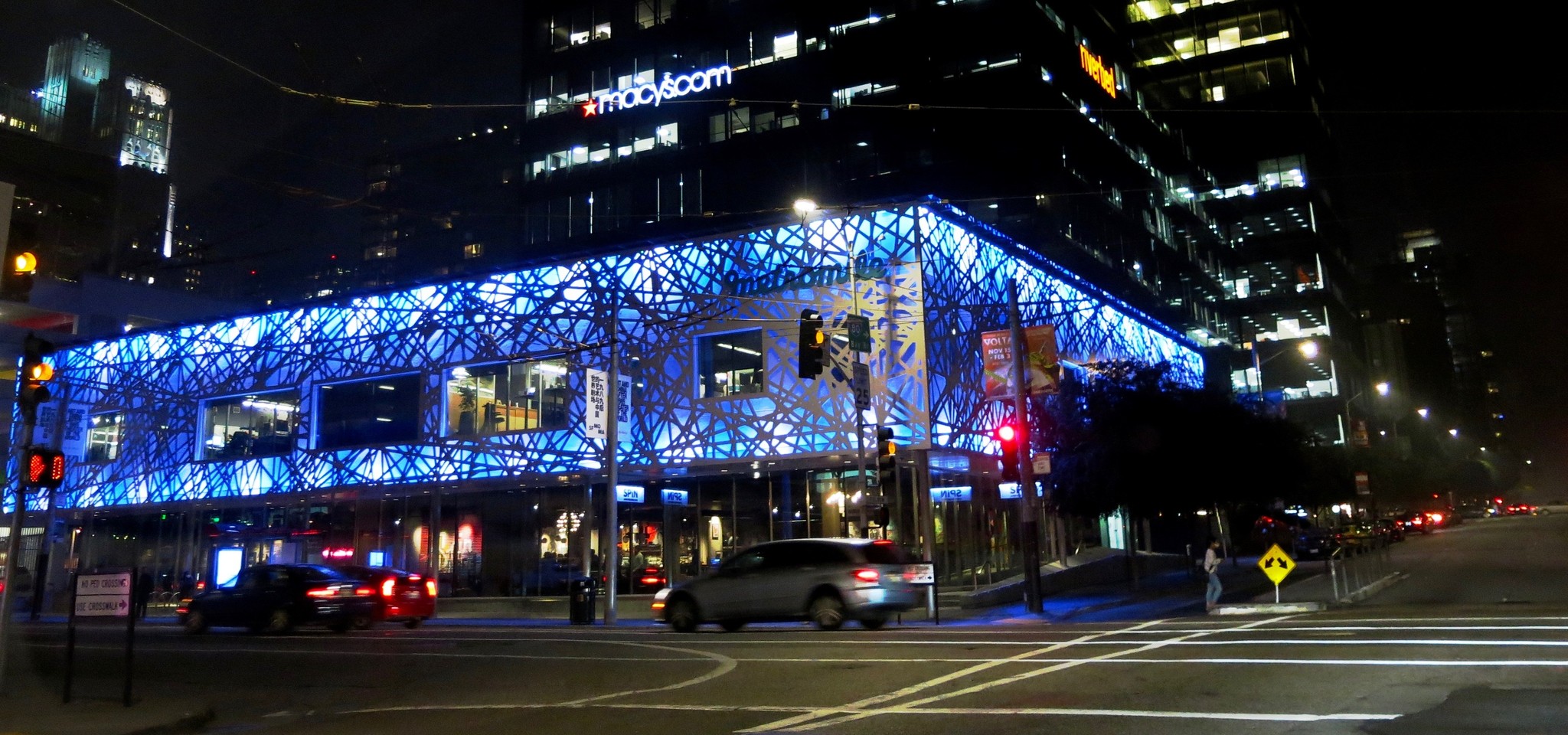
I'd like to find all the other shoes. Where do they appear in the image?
[1207,604,1221,611]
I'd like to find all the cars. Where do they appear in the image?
[179,562,373,636]
[327,564,437,631]
[1294,495,1568,559]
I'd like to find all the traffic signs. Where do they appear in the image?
[847,313,871,354]
[69,567,132,621]
[888,564,934,584]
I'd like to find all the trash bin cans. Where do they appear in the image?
[567,575,597,626]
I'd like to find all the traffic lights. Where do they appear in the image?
[1,213,42,304]
[875,423,897,486]
[18,332,56,419]
[20,448,69,489]
[797,308,824,380]
[873,505,891,526]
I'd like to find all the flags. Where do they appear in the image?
[618,373,632,443]
[585,367,608,440]
[983,330,1015,398]
[1021,324,1060,394]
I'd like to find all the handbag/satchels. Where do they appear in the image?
[1196,564,1210,583]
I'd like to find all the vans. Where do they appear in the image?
[651,537,918,631]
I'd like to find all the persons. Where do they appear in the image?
[1203,538,1225,615]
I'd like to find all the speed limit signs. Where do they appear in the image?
[851,361,871,412]
